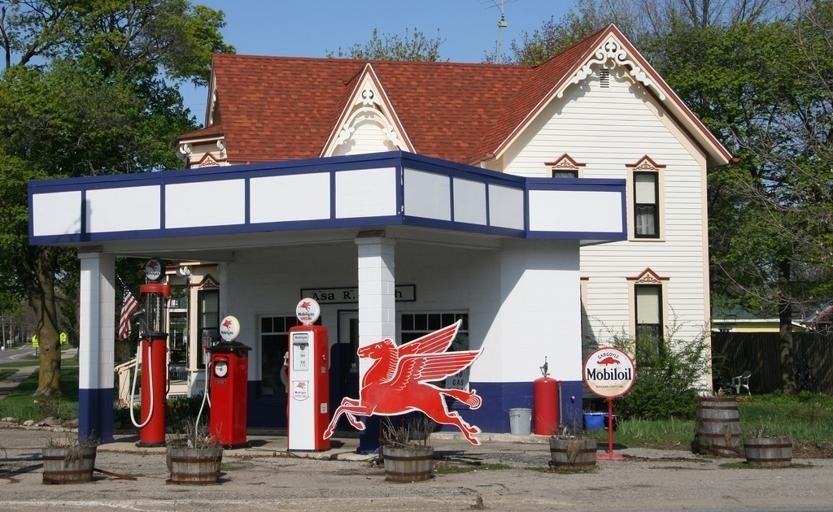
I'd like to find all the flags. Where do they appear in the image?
[118,285,140,342]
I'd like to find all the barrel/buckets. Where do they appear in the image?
[508,407,534,434]
[694,398,740,457]
[167,447,224,485]
[383,445,433,482]
[550,438,597,472]
[41,445,97,482]
[743,437,795,466]
